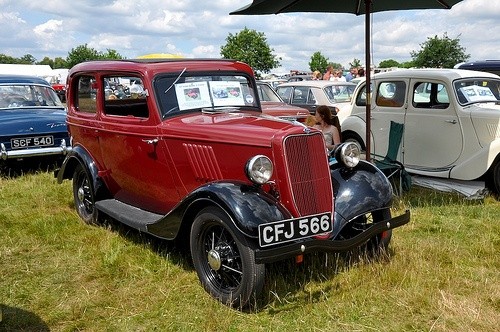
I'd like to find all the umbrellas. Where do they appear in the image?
[228,0,464,162]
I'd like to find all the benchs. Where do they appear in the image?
[105,103,149,117]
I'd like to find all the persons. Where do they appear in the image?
[311,65,364,82]
[312,105,340,153]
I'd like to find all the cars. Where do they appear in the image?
[337,67,500,200]
[0,74,73,166]
[56,56,411,307]
[238,60,500,128]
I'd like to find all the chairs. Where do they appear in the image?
[373,121,412,197]
